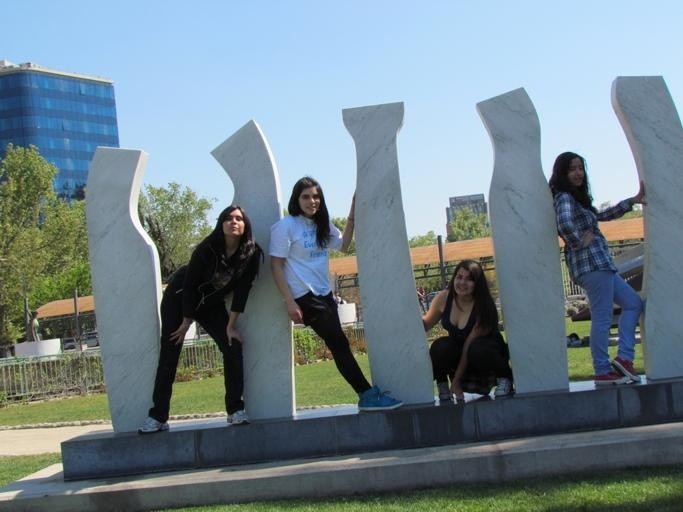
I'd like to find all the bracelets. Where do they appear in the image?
[347,215,353,220]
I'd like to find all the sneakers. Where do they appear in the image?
[135,416,170,434]
[610,355,641,382]
[493,375,512,397]
[355,386,406,413]
[437,380,453,402]
[594,372,631,386]
[225,408,249,426]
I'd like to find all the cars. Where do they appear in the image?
[422,291,438,302]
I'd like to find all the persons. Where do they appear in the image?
[137,205,263,434]
[28,311,40,341]
[549,152,647,385]
[269,176,403,411]
[417,259,512,403]
